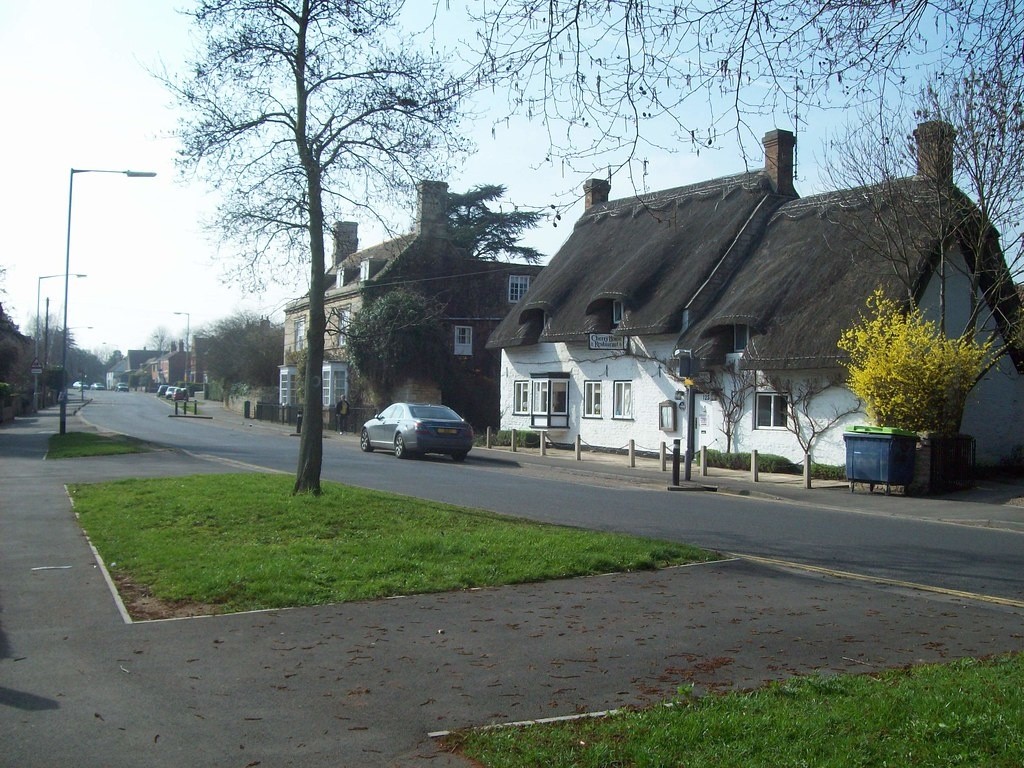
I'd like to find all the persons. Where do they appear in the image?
[336,395,350,434]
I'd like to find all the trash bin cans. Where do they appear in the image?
[842,425,921,497]
[927,434,975,495]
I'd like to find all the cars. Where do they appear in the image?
[72,382,89,389]
[157,385,170,397]
[165,386,180,399]
[115,383,130,393]
[359,402,475,462]
[173,388,189,402]
[91,383,105,390]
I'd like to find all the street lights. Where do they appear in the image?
[174,312,189,401]
[32,273,87,413]
[59,166,157,436]
